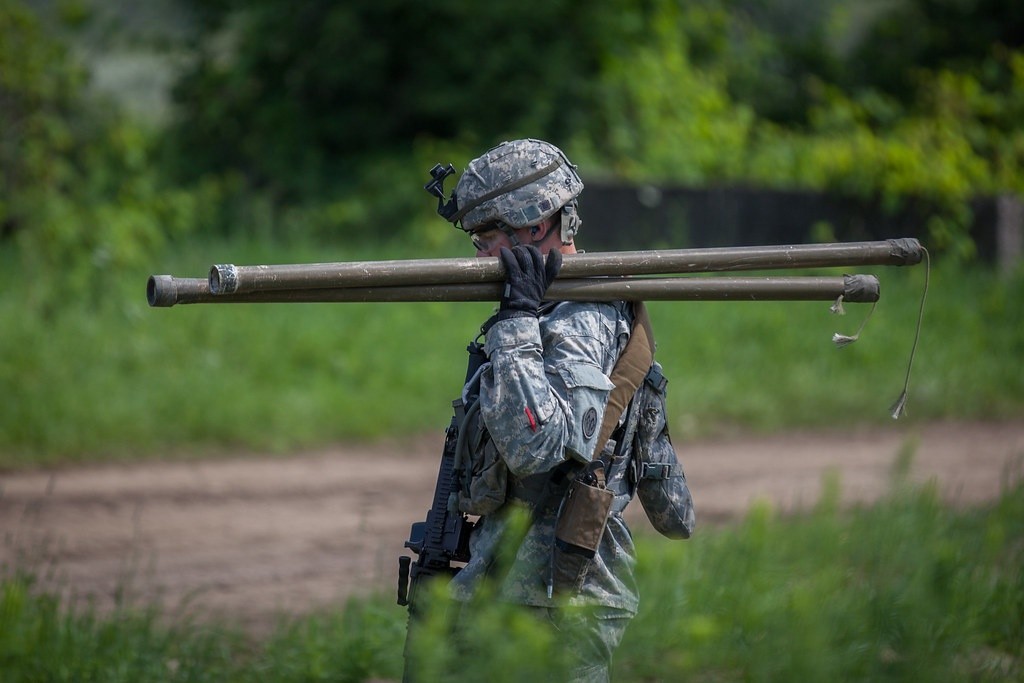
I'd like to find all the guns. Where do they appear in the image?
[395,342,493,682]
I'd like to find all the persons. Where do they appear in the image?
[445,138,656,683]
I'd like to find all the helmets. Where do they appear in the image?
[457,138,585,230]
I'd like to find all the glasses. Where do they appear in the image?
[465,224,509,253]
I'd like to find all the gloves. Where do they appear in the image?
[498,243,563,317]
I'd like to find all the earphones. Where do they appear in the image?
[531,226,540,235]
